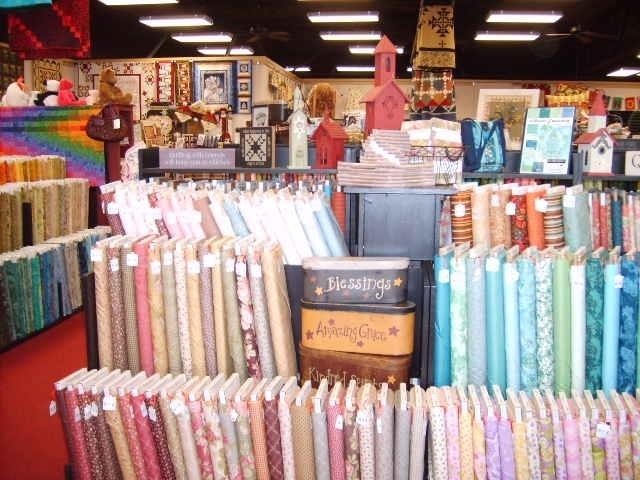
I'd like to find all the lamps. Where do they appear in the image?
[306,11,380,22]
[318,31,384,40]
[407,67,413,71]
[348,45,404,54]
[170,32,233,42]
[138,15,215,28]
[99,0,179,7]
[606,66,640,76]
[284,67,312,72]
[336,66,376,71]
[472,32,540,42]
[196,46,256,55]
[484,10,564,24]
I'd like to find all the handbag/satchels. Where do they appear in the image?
[86,116,128,142]
[459,117,506,174]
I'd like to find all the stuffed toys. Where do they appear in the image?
[2,67,132,106]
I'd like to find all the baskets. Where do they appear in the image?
[409,146,465,186]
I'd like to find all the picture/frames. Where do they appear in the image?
[92,73,142,124]
[236,96,252,114]
[237,77,252,97]
[192,59,238,114]
